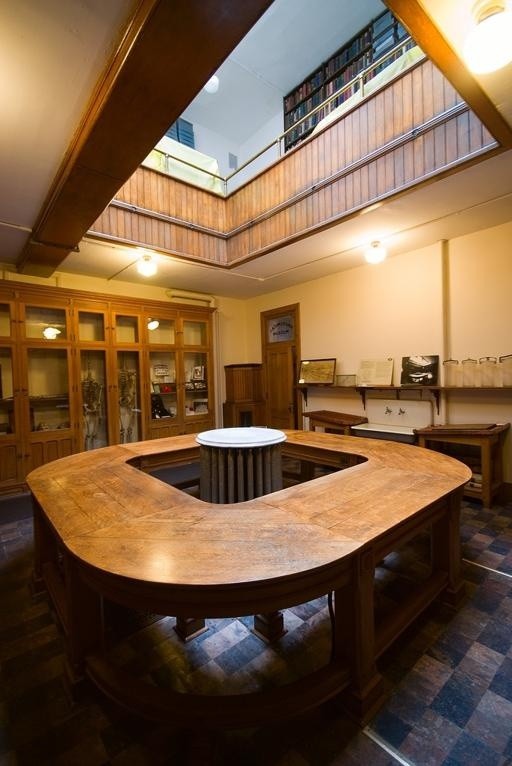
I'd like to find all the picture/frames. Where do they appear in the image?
[337,375,357,388]
[300,358,336,385]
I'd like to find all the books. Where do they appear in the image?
[281,23,376,152]
[463,471,483,490]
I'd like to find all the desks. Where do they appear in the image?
[25,429,473,739]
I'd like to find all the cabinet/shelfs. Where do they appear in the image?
[73,345,145,455]
[0,437,78,502]
[141,301,216,349]
[282,9,418,153]
[0,342,77,436]
[145,348,215,428]
[70,296,142,348]
[147,421,214,440]
[0,282,71,346]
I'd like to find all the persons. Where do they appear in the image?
[117,352,137,442]
[78,359,103,451]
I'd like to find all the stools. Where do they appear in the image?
[413,422,510,508]
[302,408,368,436]
[350,420,422,447]
